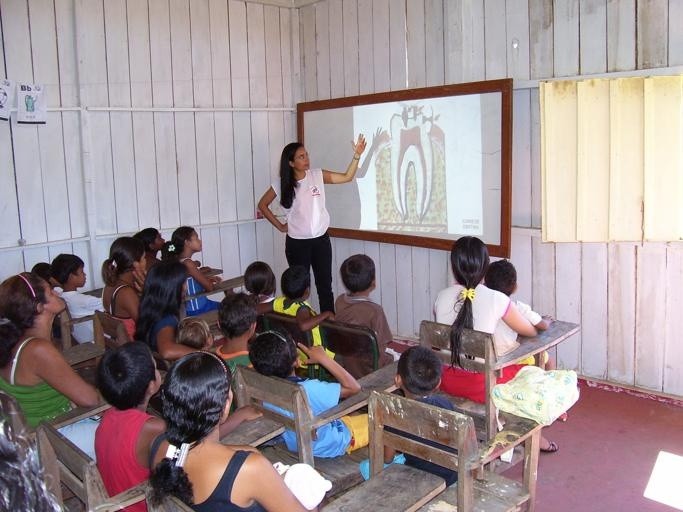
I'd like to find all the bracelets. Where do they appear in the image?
[352,156,360,160]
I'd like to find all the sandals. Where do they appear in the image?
[540,440,559,453]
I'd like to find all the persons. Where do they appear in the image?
[2,226,566,511]
[258,133,368,315]
